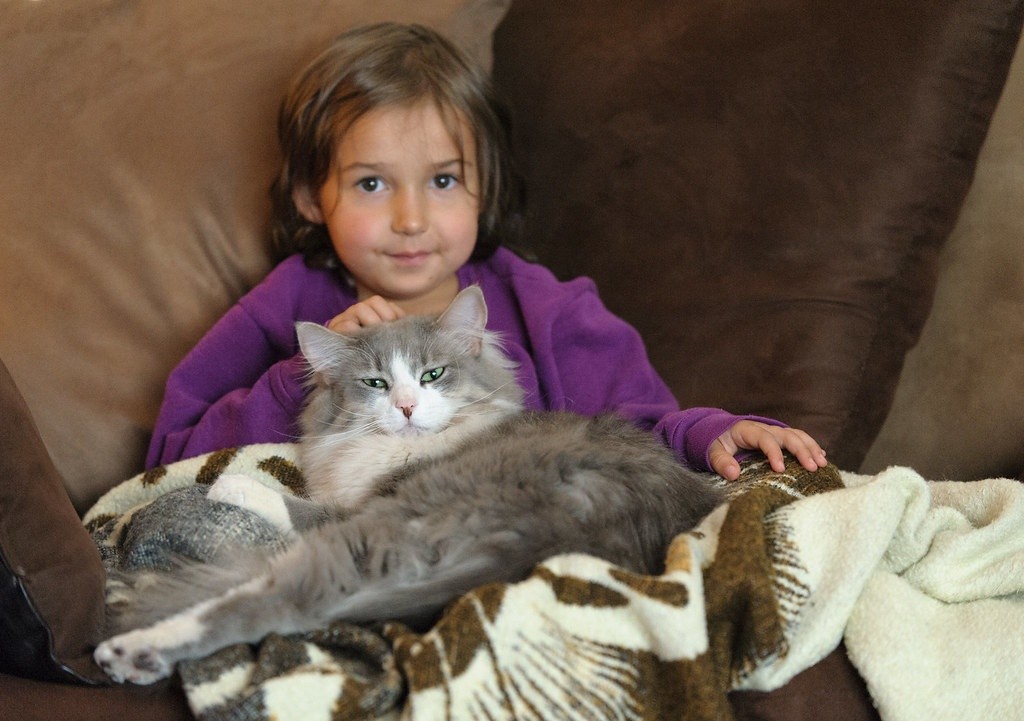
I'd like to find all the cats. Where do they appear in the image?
[94,284,724,685]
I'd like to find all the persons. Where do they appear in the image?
[148,22,829,484]
